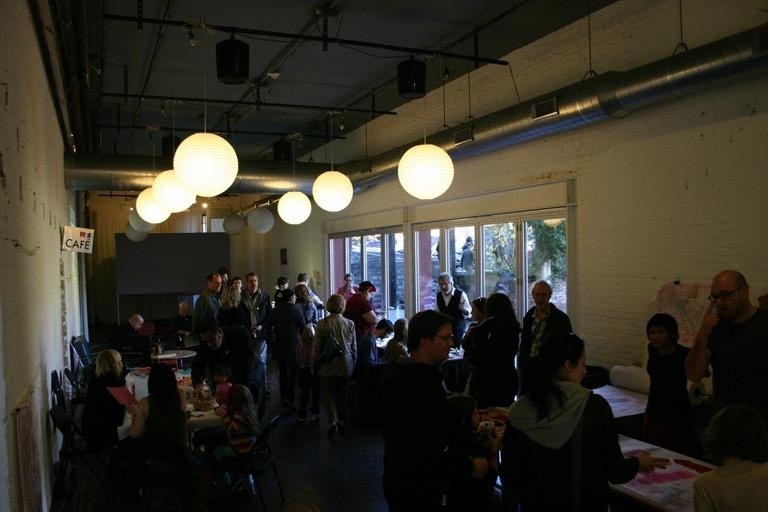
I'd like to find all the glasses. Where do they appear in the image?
[429,332,455,341]
[707,286,741,303]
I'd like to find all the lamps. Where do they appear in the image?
[277,190,312,225]
[125,223,149,240]
[312,171,354,212]
[216,38,250,84]
[136,187,171,224]
[274,139,292,162]
[152,168,198,212]
[246,208,275,234]
[396,59,426,100]
[223,214,244,234]
[160,134,180,153]
[397,143,454,201]
[129,210,156,233]
[171,131,239,198]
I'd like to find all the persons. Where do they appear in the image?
[435,273,472,349]
[685,270,768,411]
[443,393,501,512]
[313,295,357,437]
[274,288,307,406]
[169,301,192,334]
[691,403,768,512]
[463,297,489,380]
[643,314,711,448]
[294,273,323,304]
[207,382,261,498]
[345,281,376,338]
[217,288,251,329]
[519,281,573,390]
[501,330,670,512]
[382,309,465,512]
[296,324,320,424]
[468,293,519,406]
[85,349,139,440]
[209,362,235,401]
[335,273,361,303]
[242,273,272,399]
[112,314,143,345]
[385,318,412,366]
[295,284,321,324]
[358,318,393,362]
[191,273,222,323]
[191,317,267,423]
[274,279,289,301]
[127,361,187,489]
[219,270,243,289]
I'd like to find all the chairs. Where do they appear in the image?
[72,339,88,369]
[242,414,286,508]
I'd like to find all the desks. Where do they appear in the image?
[126,367,194,401]
[139,384,228,435]
[151,346,197,366]
[593,381,649,423]
[606,433,717,510]
